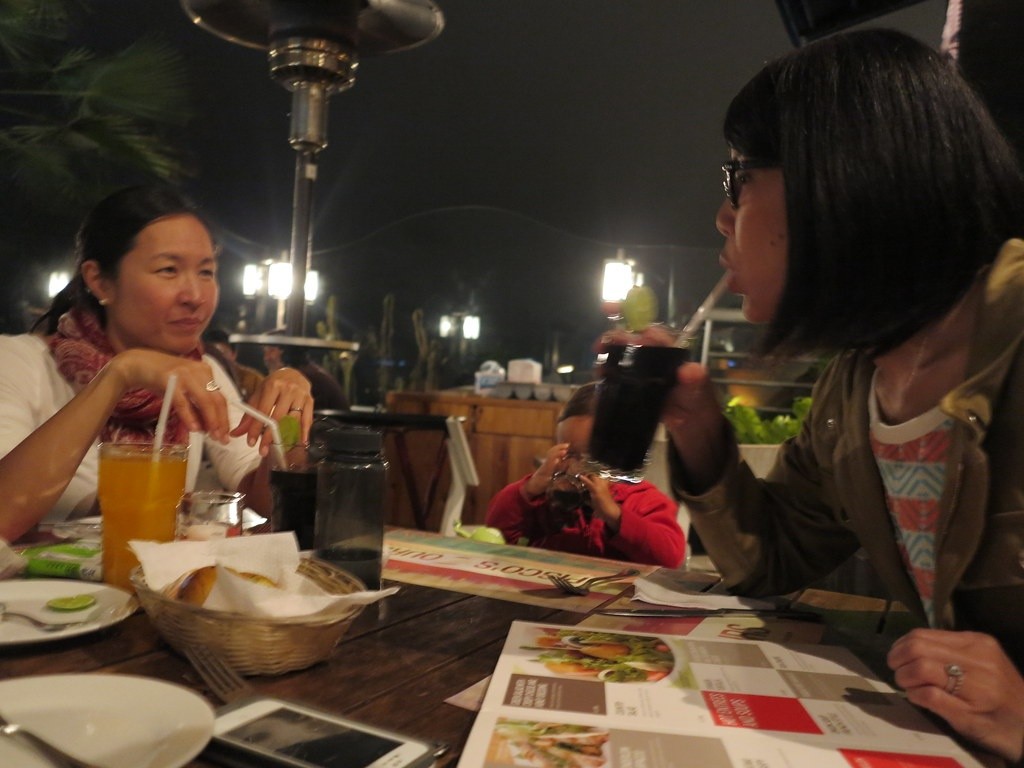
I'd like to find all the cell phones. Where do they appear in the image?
[203,694,435,768]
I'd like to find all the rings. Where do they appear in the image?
[205,380,219,392]
[291,408,303,413]
[945,663,963,695]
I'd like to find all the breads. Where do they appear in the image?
[173,565,278,608]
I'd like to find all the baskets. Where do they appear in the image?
[130,552,368,677]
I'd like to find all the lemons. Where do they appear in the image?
[623,285,659,332]
[278,414,299,452]
[47,594,96,611]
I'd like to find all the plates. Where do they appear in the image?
[0,577,139,645]
[0,672,216,767]
[53,516,102,540]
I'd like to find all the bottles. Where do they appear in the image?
[312,424,389,619]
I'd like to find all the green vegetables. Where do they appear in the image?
[532,629,672,683]
[496,718,591,768]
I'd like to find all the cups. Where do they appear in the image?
[547,453,586,508]
[172,489,250,542]
[266,443,319,557]
[589,346,689,471]
[96,441,191,594]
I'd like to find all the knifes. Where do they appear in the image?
[595,608,812,618]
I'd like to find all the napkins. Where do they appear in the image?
[631,577,766,613]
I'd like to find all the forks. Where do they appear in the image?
[546,568,640,597]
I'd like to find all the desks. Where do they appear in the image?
[311,410,463,534]
[0,529,1011,768]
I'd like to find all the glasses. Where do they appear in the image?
[720,159,786,210]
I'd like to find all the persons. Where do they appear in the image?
[590,27,1024,763]
[485,383,685,569]
[204,329,349,412]
[0,189,314,546]
